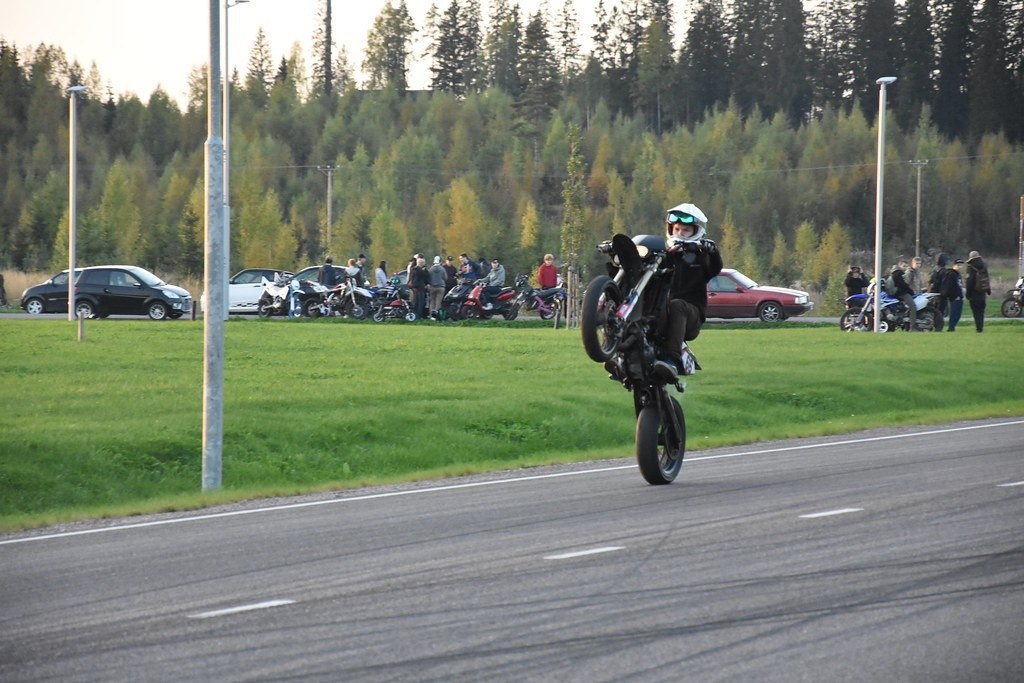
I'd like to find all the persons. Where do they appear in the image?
[900,257,921,294]
[606,203,723,384]
[476,258,505,310]
[317,255,335,286]
[965,251,992,332]
[538,254,557,290]
[374,260,389,287]
[406,252,492,320]
[928,252,949,332]
[890,261,921,332]
[343,258,364,289]
[843,264,871,323]
[356,254,366,281]
[947,259,965,332]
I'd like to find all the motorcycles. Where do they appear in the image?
[999,277,1024,319]
[840,277,945,332]
[255,273,565,322]
[581,232,701,485]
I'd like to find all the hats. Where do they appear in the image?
[433,256,441,263]
[359,254,368,259]
[446,256,454,261]
[967,251,981,263]
[954,259,964,264]
[849,267,861,274]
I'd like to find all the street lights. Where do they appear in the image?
[873,76,896,331]
[66,85,88,321]
[223,0,249,322]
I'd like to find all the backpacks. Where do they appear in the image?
[972,265,990,292]
[886,275,898,296]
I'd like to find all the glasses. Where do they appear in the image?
[669,213,693,223]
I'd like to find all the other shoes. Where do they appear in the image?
[908,321,984,333]
[486,304,493,310]
[431,316,437,321]
[427,315,432,318]
[654,361,678,385]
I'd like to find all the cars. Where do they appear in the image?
[707,268,814,321]
[258,262,368,303]
[200,269,295,313]
[74,266,193,320]
[19,268,124,314]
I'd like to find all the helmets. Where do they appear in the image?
[665,204,709,241]
